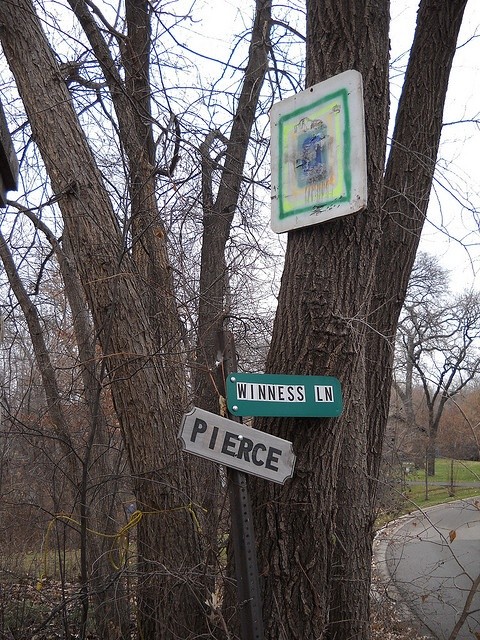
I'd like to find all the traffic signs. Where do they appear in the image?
[226,373,345,421]
[180,404,298,489]
